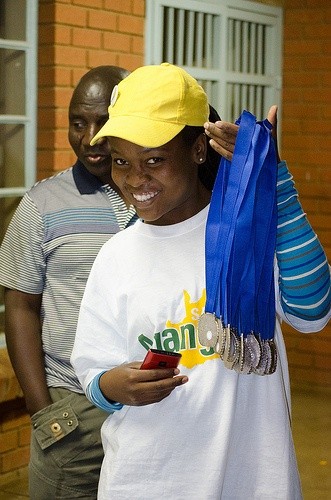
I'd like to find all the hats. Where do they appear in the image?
[89,63,209,149]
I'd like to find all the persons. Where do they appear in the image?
[70,62,331,500]
[0,65,139,500]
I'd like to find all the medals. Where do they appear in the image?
[198,313,278,376]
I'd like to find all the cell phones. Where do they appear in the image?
[140,348,182,369]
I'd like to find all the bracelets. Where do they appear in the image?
[86,371,124,412]
